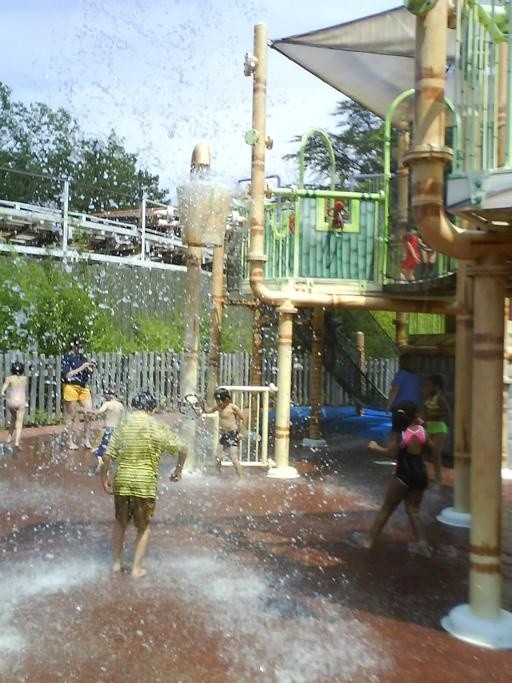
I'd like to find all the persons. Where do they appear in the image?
[386,353,429,484]
[1,362,29,453]
[417,232,437,279]
[100,392,188,580]
[350,400,437,558]
[423,373,451,493]
[60,338,95,450]
[76,389,124,474]
[396,222,422,282]
[194,388,245,482]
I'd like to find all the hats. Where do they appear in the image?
[215,388,232,401]
